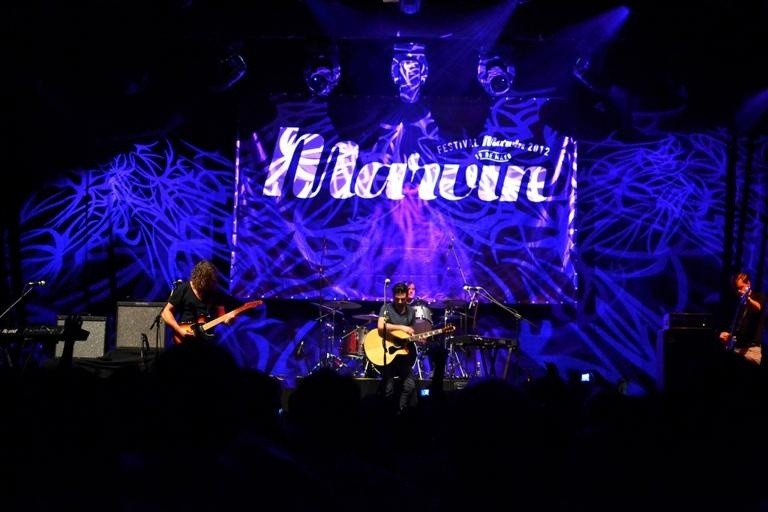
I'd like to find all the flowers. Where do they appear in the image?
[172,278,183,285]
[446,236,454,258]
[28,280,46,289]
[462,285,482,292]
[321,236,328,256]
[383,278,391,286]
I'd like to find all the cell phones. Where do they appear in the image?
[578,371,590,385]
[419,388,430,395]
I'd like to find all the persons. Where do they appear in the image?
[402,280,432,378]
[160,259,237,346]
[717,270,764,366]
[377,282,420,411]
[0,317,766,511]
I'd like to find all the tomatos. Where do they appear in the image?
[2,325,90,342]
[456,335,517,348]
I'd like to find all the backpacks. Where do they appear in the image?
[339,326,369,359]
[409,306,433,334]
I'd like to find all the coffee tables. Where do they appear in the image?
[312,303,343,314]
[353,314,379,320]
[442,300,467,306]
[331,301,361,309]
